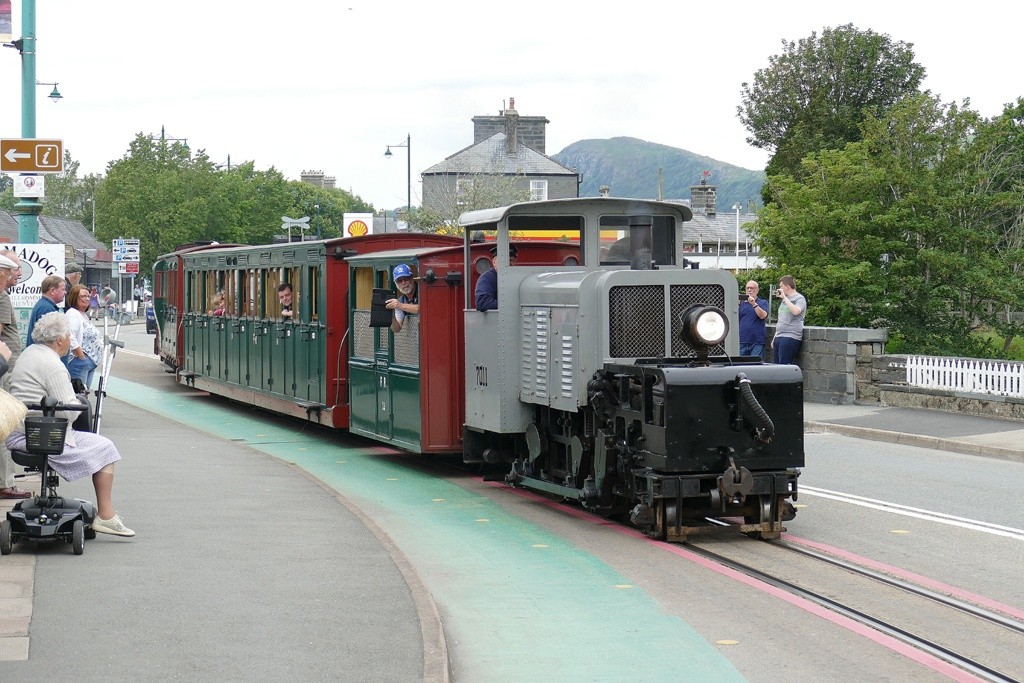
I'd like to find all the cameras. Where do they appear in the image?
[777,290,781,296]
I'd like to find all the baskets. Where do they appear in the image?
[23,415,68,455]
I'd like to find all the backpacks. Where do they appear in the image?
[90,297,97,308]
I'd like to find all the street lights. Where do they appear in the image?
[730,202,740,275]
[87,197,98,238]
[148,124,190,168]
[383,135,413,231]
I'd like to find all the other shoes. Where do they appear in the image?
[0,486,31,498]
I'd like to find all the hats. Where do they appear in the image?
[0,254,18,269]
[393,264,413,281]
[65,262,84,273]
[490,244,517,258]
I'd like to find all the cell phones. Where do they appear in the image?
[739,295,749,300]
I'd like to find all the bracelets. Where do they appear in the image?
[79,357,86,360]
[753,304,759,309]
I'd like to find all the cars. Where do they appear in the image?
[146,303,157,334]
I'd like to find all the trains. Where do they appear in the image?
[151,192,807,541]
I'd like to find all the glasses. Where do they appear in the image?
[0,270,12,280]
[396,278,409,283]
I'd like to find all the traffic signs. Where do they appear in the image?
[111,246,141,254]
[112,239,140,247]
[1,139,66,174]
[117,261,139,276]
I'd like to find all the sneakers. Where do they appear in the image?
[91,514,136,537]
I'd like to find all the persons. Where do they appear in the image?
[738,280,770,359]
[474,244,519,312]
[207,292,225,316]
[26,276,70,369]
[88,285,101,321]
[277,282,292,315]
[65,262,84,313]
[134,284,141,307]
[0,249,36,499]
[384,263,420,334]
[772,274,805,365]
[66,284,102,389]
[9,310,136,537]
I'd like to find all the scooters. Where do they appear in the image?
[1,396,102,555]
